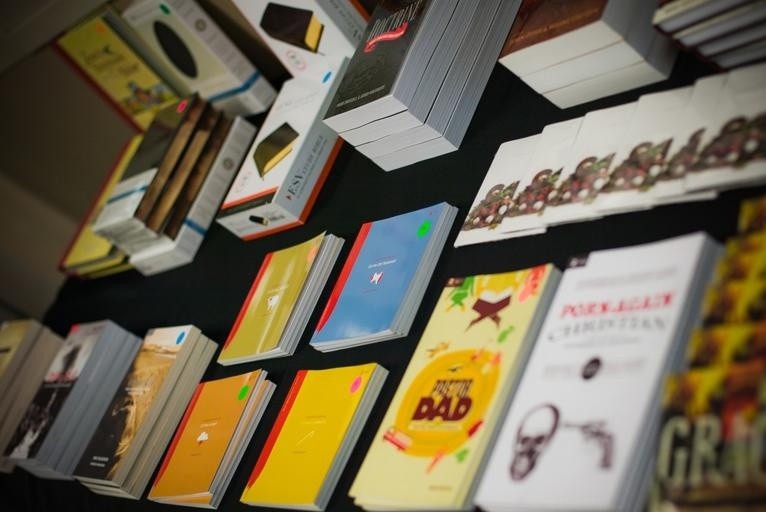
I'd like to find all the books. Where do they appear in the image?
[2,2,765,511]
